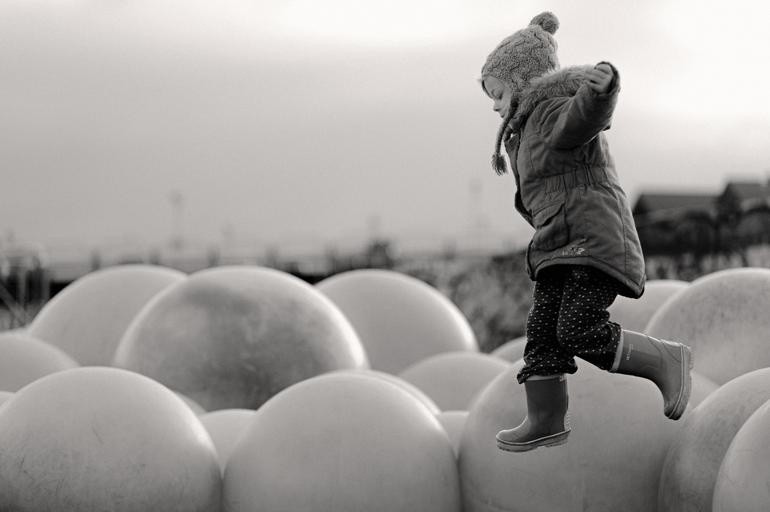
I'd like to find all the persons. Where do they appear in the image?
[479,13,692,451]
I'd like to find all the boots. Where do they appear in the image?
[494,372,572,453]
[608,328,694,423]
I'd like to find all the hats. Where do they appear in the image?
[479,10,562,106]
